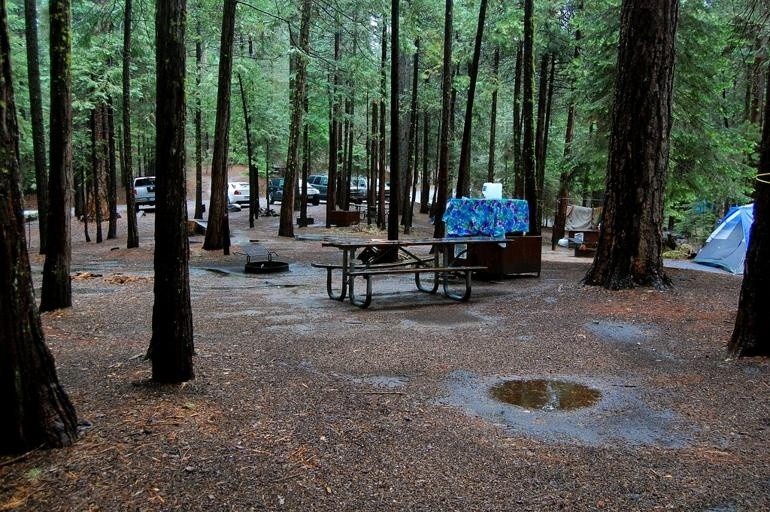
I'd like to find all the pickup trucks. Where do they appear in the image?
[133,174,157,212]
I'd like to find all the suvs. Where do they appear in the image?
[307,172,391,203]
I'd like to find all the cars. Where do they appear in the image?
[228,182,250,203]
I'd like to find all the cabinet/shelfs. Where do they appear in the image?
[329,209,361,227]
[443,232,544,282]
[566,230,602,250]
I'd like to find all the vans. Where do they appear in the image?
[268,177,322,206]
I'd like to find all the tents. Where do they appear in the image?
[690,201,756,275]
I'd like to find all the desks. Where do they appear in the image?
[444,197,533,238]
[310,236,516,309]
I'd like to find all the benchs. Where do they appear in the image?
[345,264,490,310]
[311,256,440,303]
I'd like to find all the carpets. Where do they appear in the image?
[662,258,736,276]
